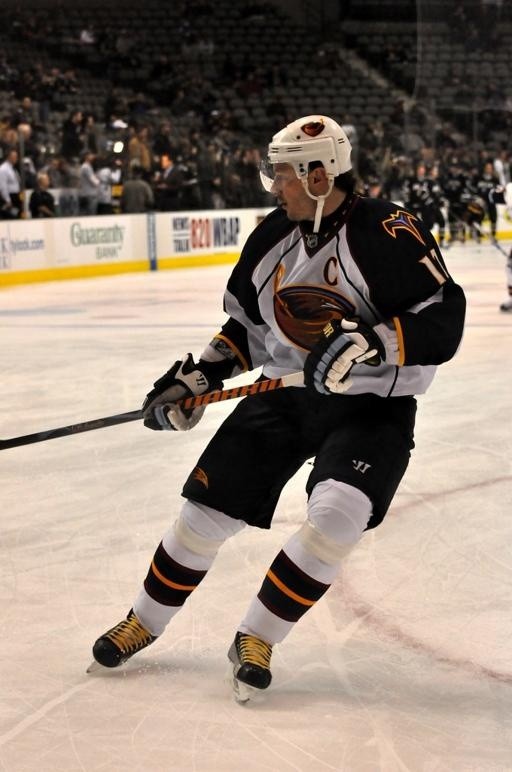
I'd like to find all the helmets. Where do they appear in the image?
[259,115,353,194]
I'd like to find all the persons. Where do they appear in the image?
[0,0,511,249]
[92,111,468,690]
[497,183,511,314]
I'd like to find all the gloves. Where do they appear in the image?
[303,317,405,398]
[142,352,224,431]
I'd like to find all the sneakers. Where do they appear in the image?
[92,608,158,667]
[234,631,272,690]
[500,301,512,311]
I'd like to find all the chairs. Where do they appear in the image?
[2,3,512,190]
